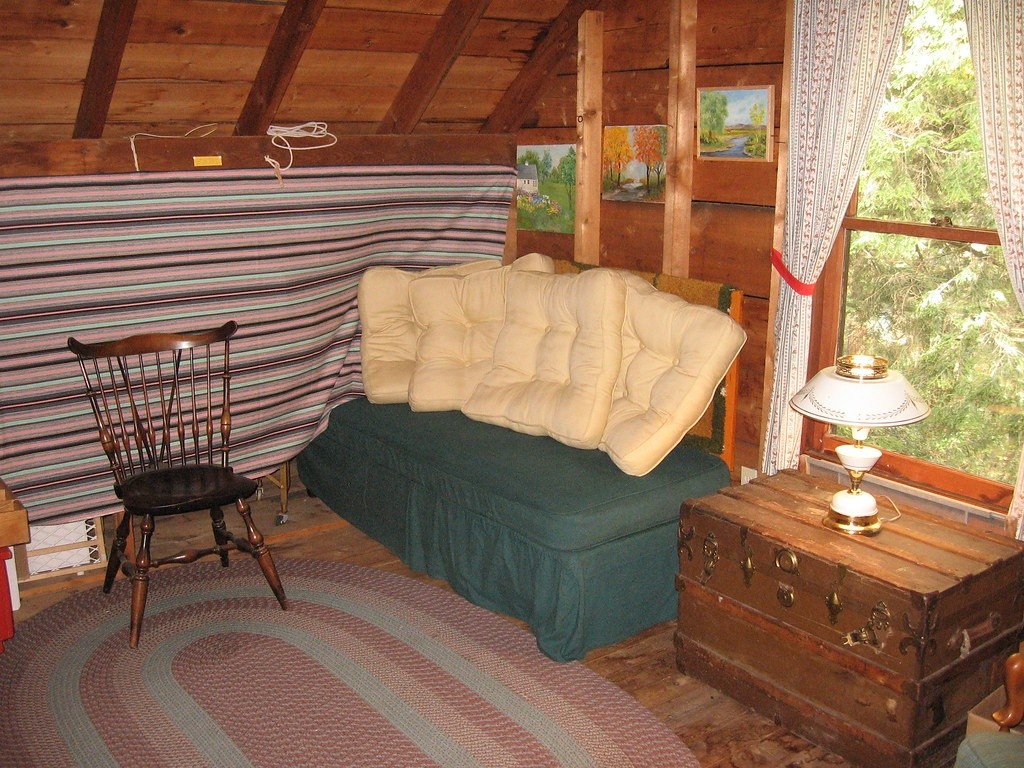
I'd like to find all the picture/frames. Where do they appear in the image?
[696,85,774,163]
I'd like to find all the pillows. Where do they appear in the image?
[465,268,626,452]
[599,270,749,478]
[356,256,500,402]
[408,251,555,412]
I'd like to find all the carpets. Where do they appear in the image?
[0,555,702,768]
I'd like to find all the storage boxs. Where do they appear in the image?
[965,679,1024,738]
[674,464,1024,768]
[0,477,31,549]
[12,516,108,583]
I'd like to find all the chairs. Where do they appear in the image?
[65,320,290,648]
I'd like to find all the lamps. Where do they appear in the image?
[790,351,936,536]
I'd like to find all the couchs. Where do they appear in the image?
[297,258,744,666]
